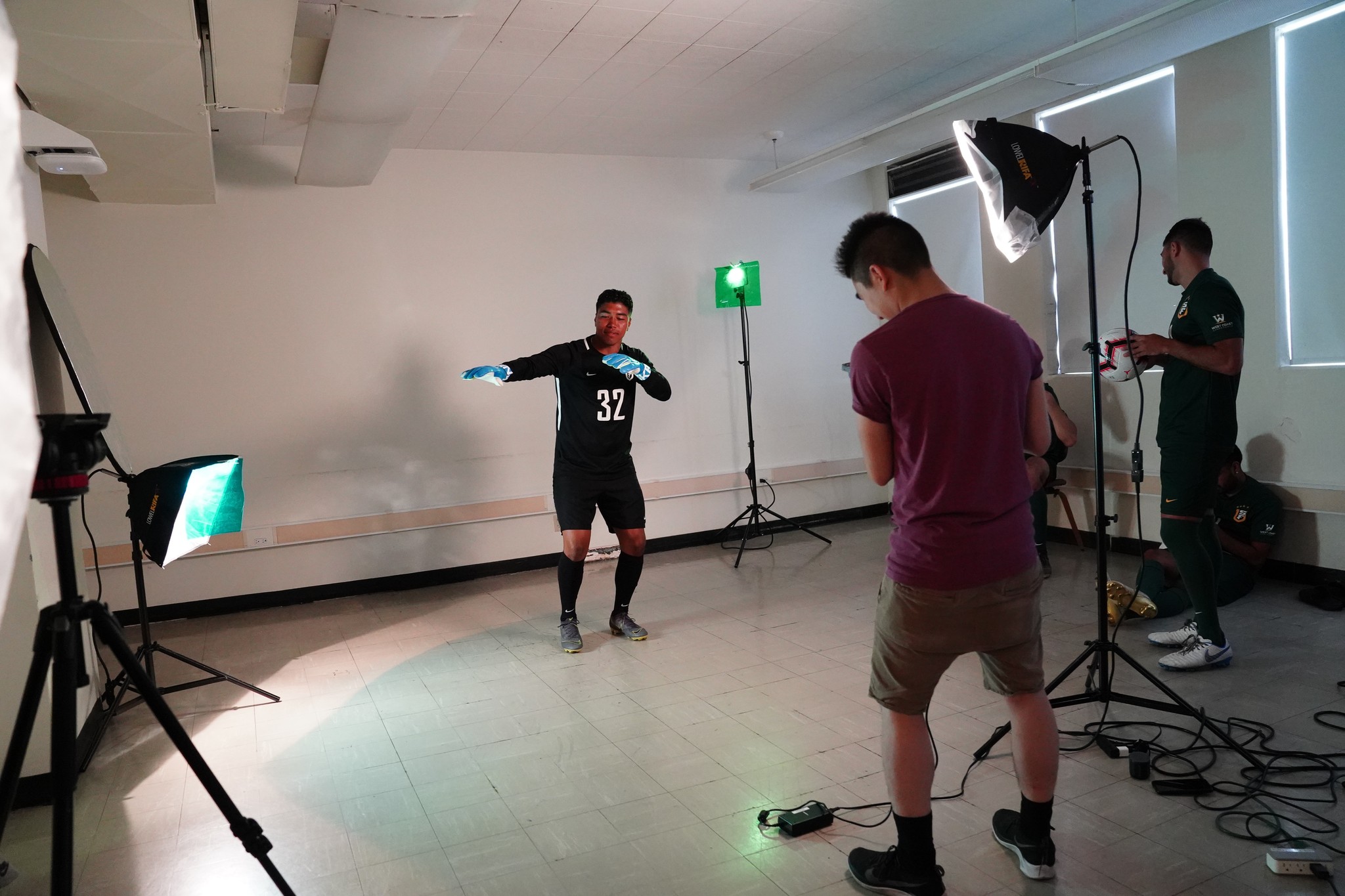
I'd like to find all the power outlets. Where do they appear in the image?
[243,527,278,548]
[756,469,772,486]
[547,495,556,511]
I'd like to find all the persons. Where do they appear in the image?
[835,213,1060,896]
[460,288,671,656]
[1121,219,1246,667]
[1096,443,1284,627]
[1024,383,1076,577]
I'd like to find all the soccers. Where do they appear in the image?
[1098,327,1148,382]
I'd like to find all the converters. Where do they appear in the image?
[777,802,834,837]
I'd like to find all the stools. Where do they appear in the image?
[1048,478,1084,551]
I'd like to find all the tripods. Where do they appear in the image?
[0,414,297,896]
[706,291,832,568]
[973,136,1269,773]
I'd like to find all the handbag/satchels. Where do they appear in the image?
[1297,583,1345,611]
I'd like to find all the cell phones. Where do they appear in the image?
[1151,778,1213,795]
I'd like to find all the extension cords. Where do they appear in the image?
[1266,848,1335,877]
[1094,735,1120,759]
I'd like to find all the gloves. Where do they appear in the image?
[461,364,513,386]
[602,353,651,381]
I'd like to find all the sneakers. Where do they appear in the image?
[608,610,648,641]
[1148,619,1199,648]
[992,808,1055,879]
[557,615,583,653]
[1158,635,1233,670]
[846,844,948,896]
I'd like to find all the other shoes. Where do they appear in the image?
[1106,581,1158,618]
[1036,542,1051,578]
[1095,579,1124,625]
